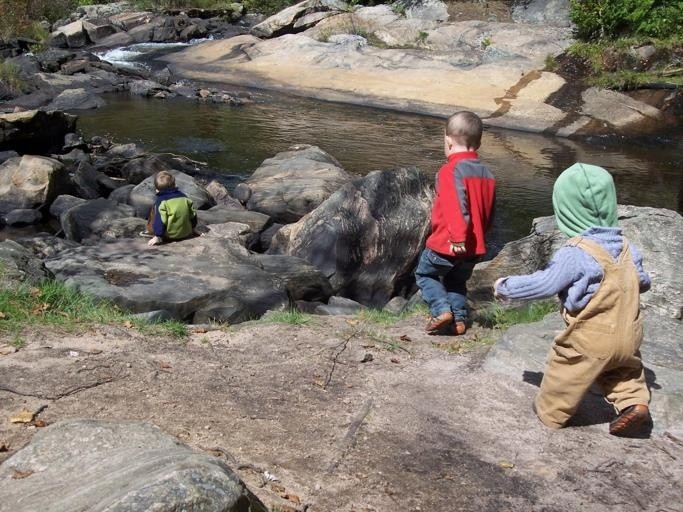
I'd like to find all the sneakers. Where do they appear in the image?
[139,231,154,238]
[445,320,467,335]
[609,404,651,437]
[425,311,455,332]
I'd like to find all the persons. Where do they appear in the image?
[413,109,496,335]
[490,161,652,436]
[138,170,198,246]
